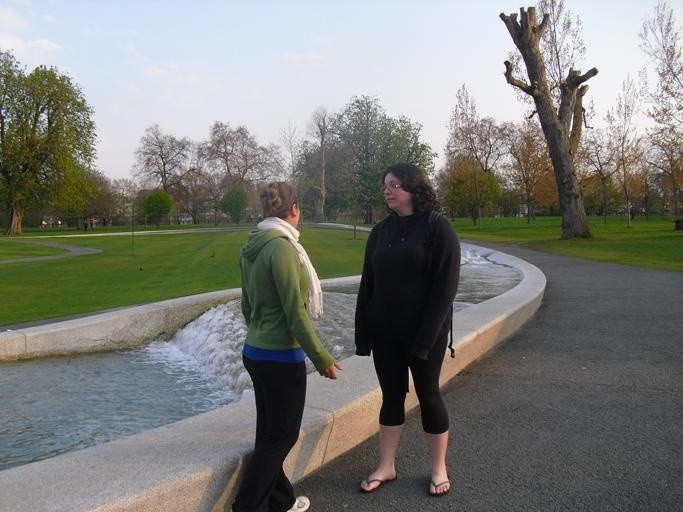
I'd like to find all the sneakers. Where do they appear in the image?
[285,495,310,512]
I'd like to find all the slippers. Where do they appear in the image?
[428,470,452,496]
[359,470,398,494]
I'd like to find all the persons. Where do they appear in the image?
[353,162,461,496]
[229,180,344,511]
[82,216,97,233]
